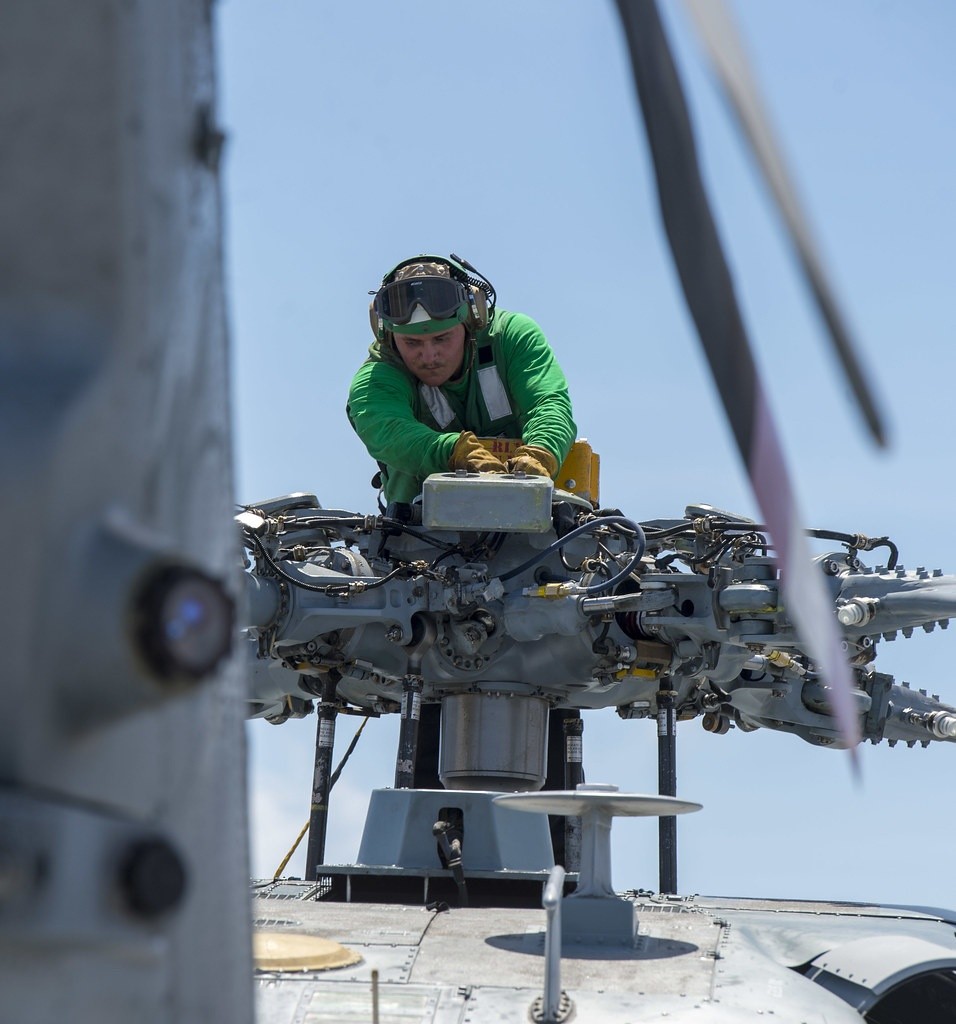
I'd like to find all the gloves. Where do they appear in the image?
[504,445,558,477]
[448,430,508,473]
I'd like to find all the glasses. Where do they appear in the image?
[374,275,466,324]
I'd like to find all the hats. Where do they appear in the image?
[382,263,468,334]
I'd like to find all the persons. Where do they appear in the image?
[347,256,578,514]
